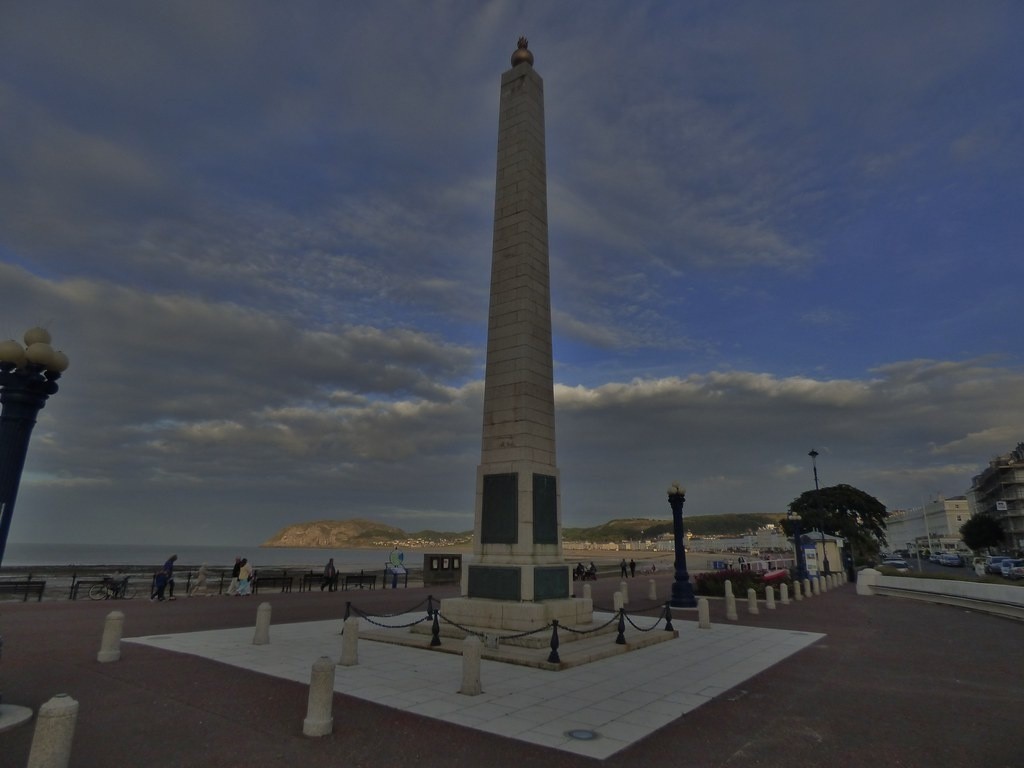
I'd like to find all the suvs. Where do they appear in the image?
[985,556,1008,574]
[1001,558,1024,579]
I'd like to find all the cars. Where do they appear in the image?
[883,558,908,569]
[971,556,988,571]
[928,553,960,567]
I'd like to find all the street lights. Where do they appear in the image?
[0,326,73,571]
[808,449,831,574]
[666,481,697,607]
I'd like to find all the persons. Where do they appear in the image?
[629,559,636,578]
[724,558,733,569]
[321,558,335,592]
[652,564,655,575]
[576,561,597,581]
[224,555,255,596]
[620,559,628,579]
[149,554,178,600]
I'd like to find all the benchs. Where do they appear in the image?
[252,577,293,593]
[0,581,47,601]
[299,574,338,592]
[69,580,128,600]
[342,575,377,590]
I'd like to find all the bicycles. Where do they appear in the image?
[88,575,138,601]
